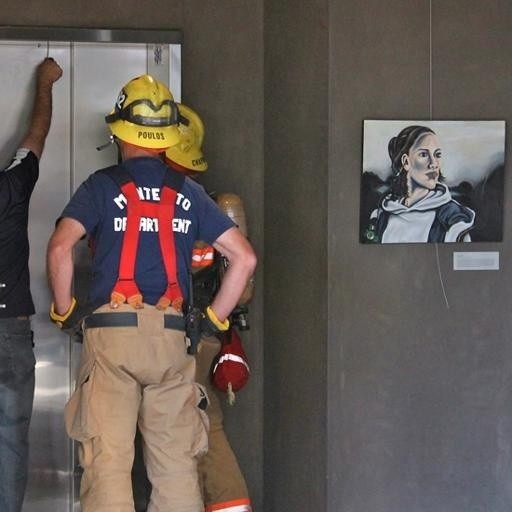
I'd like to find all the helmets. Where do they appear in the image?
[164,102,209,172]
[105,74,182,149]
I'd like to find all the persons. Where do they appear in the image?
[0,57,63,512]
[161,102,254,512]
[46,73,257,512]
[361,126,477,243]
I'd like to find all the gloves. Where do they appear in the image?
[199,305,230,338]
[49,296,93,344]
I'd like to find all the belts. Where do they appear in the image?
[84,312,187,332]
